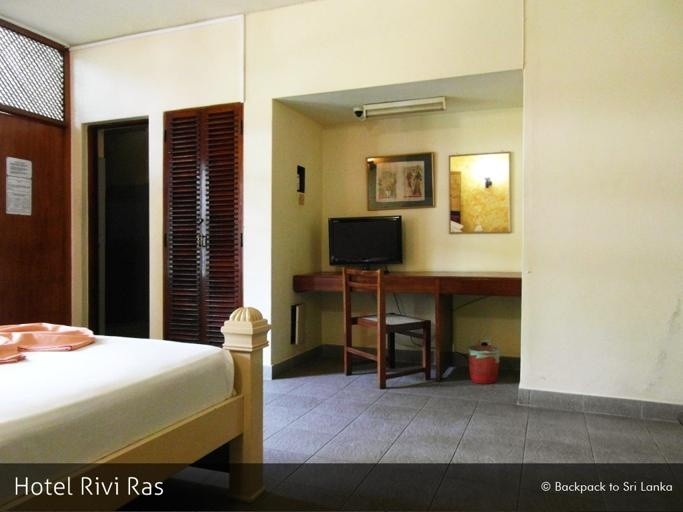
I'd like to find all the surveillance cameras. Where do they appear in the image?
[352,106,364,119]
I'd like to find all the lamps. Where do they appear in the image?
[362,96,446,120]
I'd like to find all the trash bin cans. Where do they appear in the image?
[469,344,500,384]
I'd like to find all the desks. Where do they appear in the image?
[294,268,521,383]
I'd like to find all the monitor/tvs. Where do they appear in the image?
[328,215,404,274]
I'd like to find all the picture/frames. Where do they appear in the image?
[365,151,436,212]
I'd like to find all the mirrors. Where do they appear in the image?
[446,151,511,234]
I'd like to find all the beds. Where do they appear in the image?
[0,306,273,511]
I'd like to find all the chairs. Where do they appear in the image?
[340,267,432,390]
